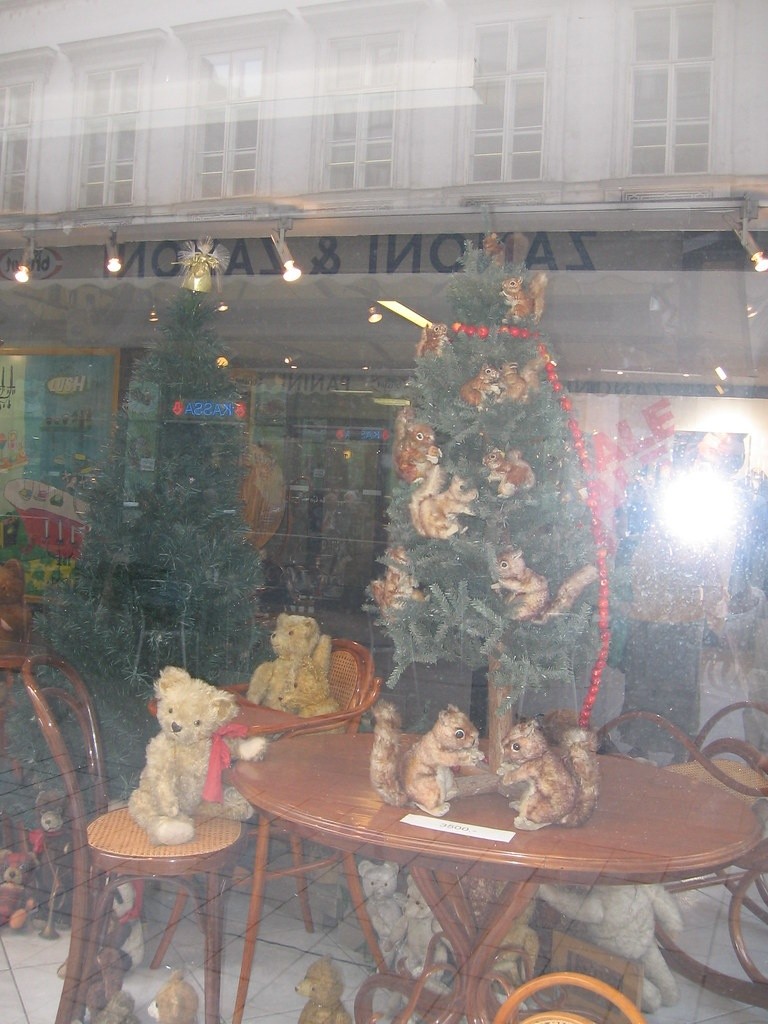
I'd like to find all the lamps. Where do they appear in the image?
[270,218,301,282]
[14,238,34,282]
[104,227,121,272]
[735,193,768,272]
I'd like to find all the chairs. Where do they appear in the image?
[23,657,246,1023]
[599,702,768,1010]
[492,973,645,1024]
[147,639,379,928]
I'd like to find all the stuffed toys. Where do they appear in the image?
[0,558,34,701]
[371,233,597,831]
[0,788,686,1024]
[245,613,346,737]
[126,665,255,846]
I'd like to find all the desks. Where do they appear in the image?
[229,733,768,1024]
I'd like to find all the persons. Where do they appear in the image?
[610,500,727,766]
